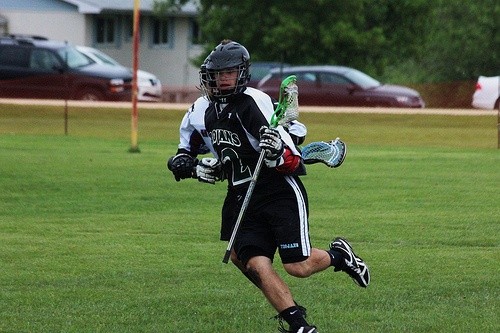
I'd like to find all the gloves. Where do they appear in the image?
[172,153,195,182]
[195,158,220,184]
[259,126,285,161]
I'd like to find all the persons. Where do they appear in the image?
[171,41,372,333]
[167,53,307,320]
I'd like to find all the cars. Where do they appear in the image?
[256,66,425,108]
[471,76,500,109]
[0,33,161,101]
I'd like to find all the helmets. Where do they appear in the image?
[200,40,253,104]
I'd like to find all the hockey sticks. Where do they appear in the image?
[301,137,347,169]
[222,75,299,264]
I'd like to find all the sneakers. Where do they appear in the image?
[287,324,317,333]
[329,237,371,287]
